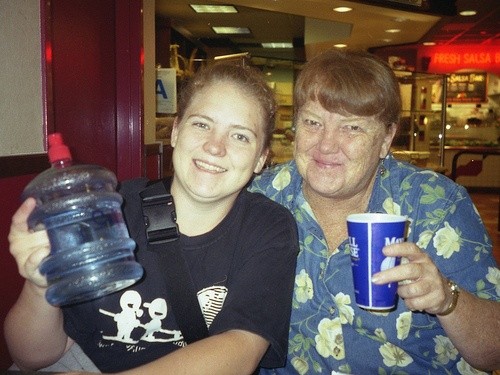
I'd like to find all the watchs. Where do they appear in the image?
[437,279,461,316]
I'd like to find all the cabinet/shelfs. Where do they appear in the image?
[389,74,447,173]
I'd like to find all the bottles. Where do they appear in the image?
[21,132,143,306]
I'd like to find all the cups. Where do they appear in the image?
[347,213,408,310]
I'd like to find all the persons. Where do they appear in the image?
[3,59,301,375]
[245,47,500,375]
